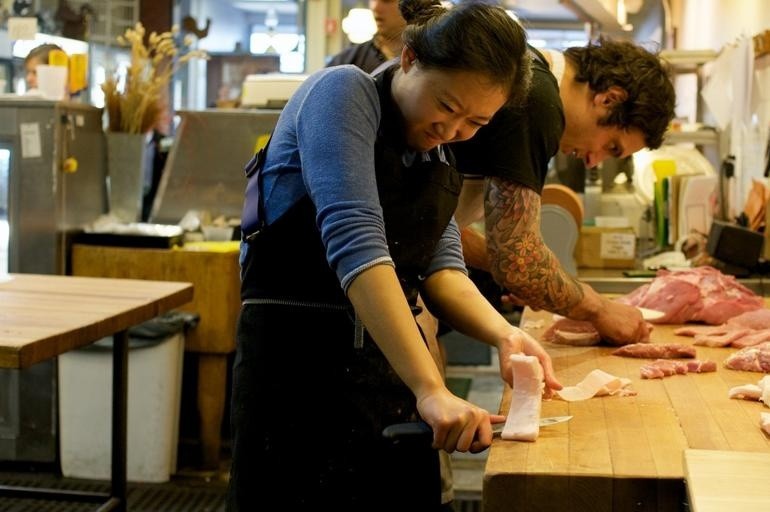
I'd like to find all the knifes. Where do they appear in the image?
[384,414,574,451]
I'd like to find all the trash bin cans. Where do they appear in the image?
[58,308,201,483]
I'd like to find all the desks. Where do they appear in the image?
[0,240,239,512]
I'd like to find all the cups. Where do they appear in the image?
[36,65,69,102]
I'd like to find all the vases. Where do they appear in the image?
[105,128,147,223]
